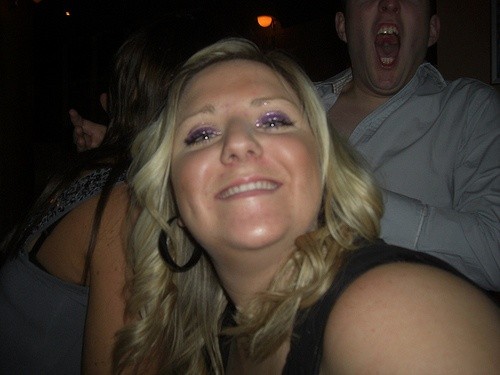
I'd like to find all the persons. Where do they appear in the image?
[67,0,500,294]
[0,11,244,375]
[108,37,500,375]
